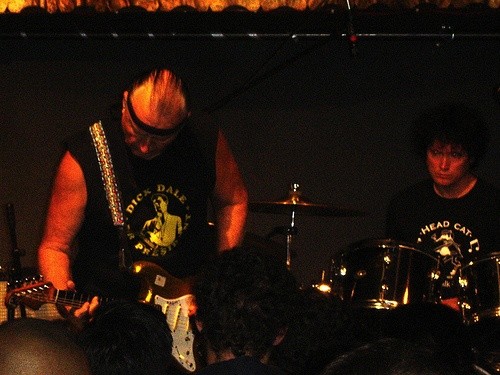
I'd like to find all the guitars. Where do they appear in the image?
[5,261,204,374]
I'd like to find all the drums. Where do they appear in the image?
[458,249,500,326]
[329,237,448,312]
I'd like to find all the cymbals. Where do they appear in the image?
[246,192,372,218]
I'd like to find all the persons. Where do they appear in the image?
[0,318,94,375]
[270,288,500,375]
[77,299,175,375]
[37,69,248,319]
[192,247,298,375]
[386,101,500,311]
